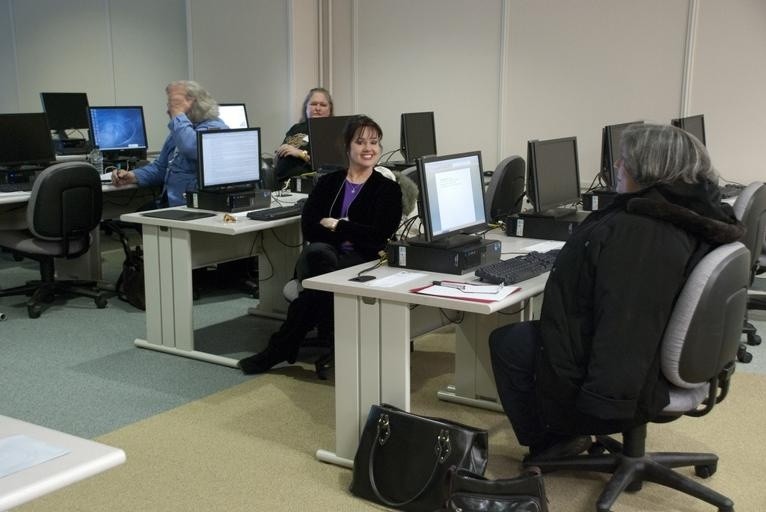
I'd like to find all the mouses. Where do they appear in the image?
[297,198,309,204]
[546,249,563,254]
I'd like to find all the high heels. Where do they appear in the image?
[238,334,298,374]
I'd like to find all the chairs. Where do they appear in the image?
[484,156,524,222]
[732,180,766,364]
[1,161,114,318]
[524,241,751,510]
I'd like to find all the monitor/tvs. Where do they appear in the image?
[517,135,583,219]
[671,113,710,151]
[196,126,264,196]
[88,106,150,161]
[599,120,645,189]
[398,112,436,161]
[0,112,58,172]
[307,114,363,174]
[38,91,92,141]
[416,149,490,250]
[203,103,250,133]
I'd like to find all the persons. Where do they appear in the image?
[237,116,402,375]
[489,124,748,472]
[245,89,333,299]
[112,81,231,300]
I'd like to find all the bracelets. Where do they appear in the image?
[302,150,308,161]
[331,220,340,232]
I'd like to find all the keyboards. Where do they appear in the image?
[473,251,558,284]
[712,186,744,199]
[245,204,306,220]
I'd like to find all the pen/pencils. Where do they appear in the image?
[116,163,121,184]
[433,281,465,289]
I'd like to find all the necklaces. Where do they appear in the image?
[349,171,372,193]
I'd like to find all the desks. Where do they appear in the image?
[0,415,126,511]
[0,168,136,293]
[302,183,747,468]
[120,163,419,366]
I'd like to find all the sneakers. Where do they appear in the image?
[522,433,592,463]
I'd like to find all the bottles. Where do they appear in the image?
[89,145,104,177]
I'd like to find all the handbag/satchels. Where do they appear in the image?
[348,403,489,512]
[444,464,550,512]
[115,250,144,311]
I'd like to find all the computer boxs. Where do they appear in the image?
[504,210,595,242]
[50,138,93,154]
[1,166,50,187]
[378,160,421,179]
[89,154,149,171]
[291,172,336,195]
[186,190,272,214]
[584,192,630,212]
[387,235,504,275]
